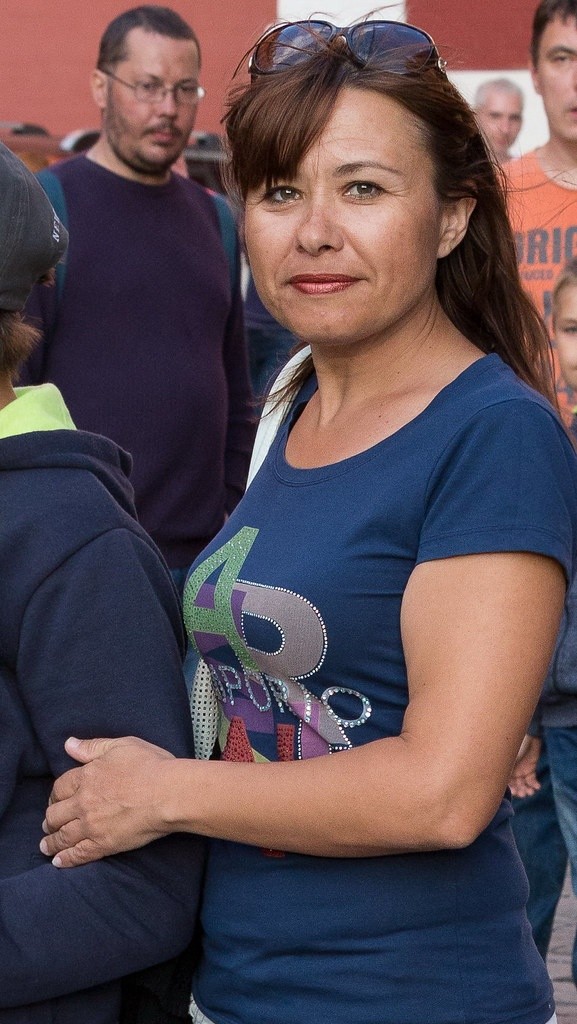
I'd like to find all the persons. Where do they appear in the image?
[2,0,576,984]
[40,19,577,1024]
[1,141,200,1024]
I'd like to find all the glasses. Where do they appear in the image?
[102,68,205,104]
[248,20,448,85]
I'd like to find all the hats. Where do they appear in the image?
[0,141,68,311]
